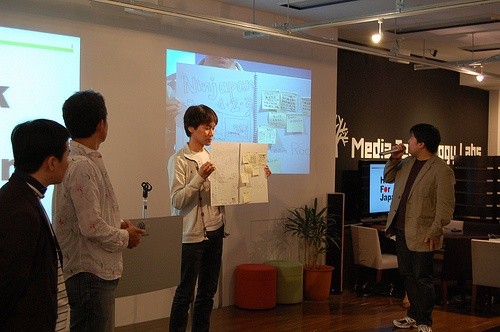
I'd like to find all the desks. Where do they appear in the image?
[438,226,492,243]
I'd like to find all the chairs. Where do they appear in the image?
[471,238,500,306]
[351,224,398,285]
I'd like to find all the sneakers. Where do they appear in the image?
[392,316,417,328]
[417,325,432,332]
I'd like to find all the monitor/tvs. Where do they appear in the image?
[358,160,395,219]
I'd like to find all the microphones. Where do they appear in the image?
[379,146,406,155]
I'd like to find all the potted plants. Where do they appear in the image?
[281,199,335,302]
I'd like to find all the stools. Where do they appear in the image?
[234,259,303,310]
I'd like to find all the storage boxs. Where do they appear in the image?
[420,277,457,306]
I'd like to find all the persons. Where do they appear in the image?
[383,122,456,332]
[0,119,71,332]
[50,89,146,332]
[164,54,245,117]
[166,104,272,332]
